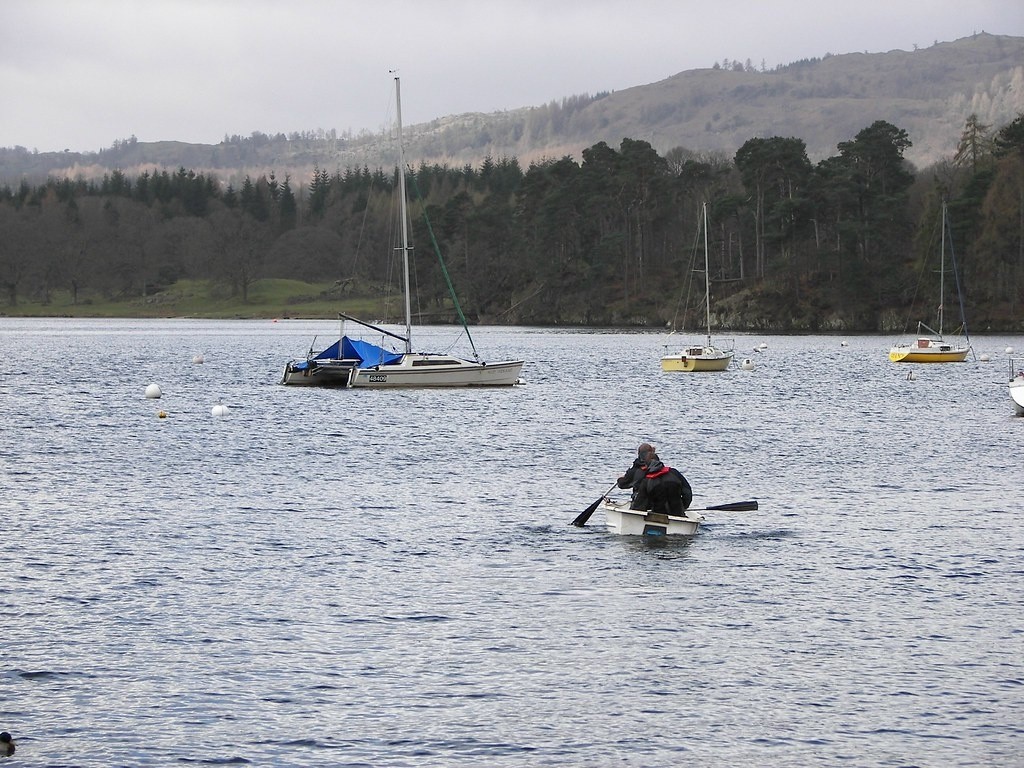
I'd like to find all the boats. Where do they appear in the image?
[1007,363,1024,417]
[605,502,703,537]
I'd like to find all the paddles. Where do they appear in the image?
[571,482,618,525]
[684,500,759,512]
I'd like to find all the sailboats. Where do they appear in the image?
[890,193,972,363]
[660,202,737,373]
[280,76,525,390]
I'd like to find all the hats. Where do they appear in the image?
[638,443,655,453]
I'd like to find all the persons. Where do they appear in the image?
[617,443,692,517]
